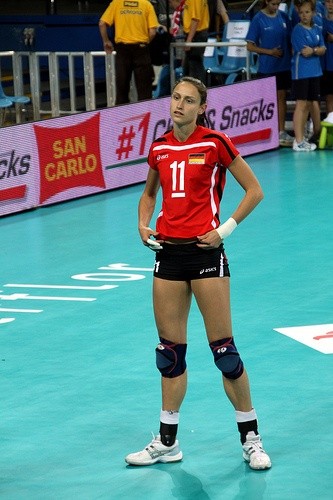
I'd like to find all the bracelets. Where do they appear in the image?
[312,46,318,55]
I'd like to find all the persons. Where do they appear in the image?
[289,0,322,151]
[288,0,332,140]
[98,0,160,105]
[148,0,229,88]
[244,0,295,147]
[125,76,272,470]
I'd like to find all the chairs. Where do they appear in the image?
[151,3,288,99]
[1,84,30,123]
[0,98,14,127]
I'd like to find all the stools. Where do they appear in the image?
[319,121,333,149]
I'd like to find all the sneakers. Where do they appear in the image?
[241,430,271,469]
[279,131,298,146]
[293,139,317,151]
[124,434,183,466]
